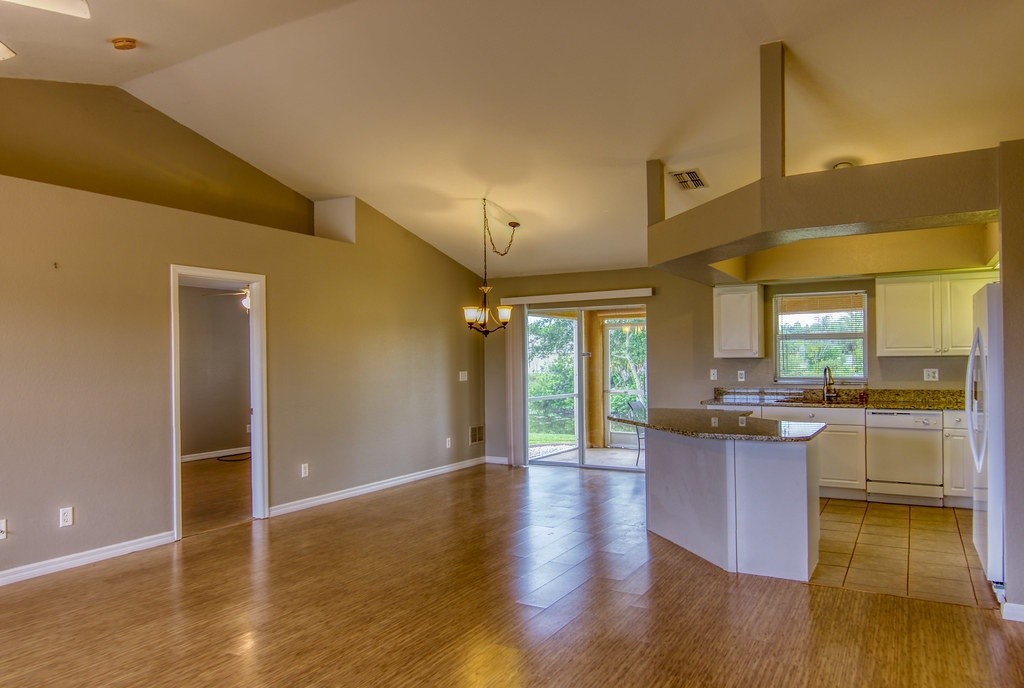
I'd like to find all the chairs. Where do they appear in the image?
[628,401,646,466]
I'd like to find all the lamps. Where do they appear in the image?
[461,197,520,338]
[241,293,249,314]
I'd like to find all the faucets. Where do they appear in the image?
[824,365,834,385]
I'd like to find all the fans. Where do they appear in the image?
[202,284,249,296]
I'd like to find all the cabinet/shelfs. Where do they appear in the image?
[942,409,976,498]
[874,267,1000,357]
[761,406,866,490]
[713,284,765,359]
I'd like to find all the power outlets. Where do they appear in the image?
[59,506,72,527]
[0,520,7,540]
